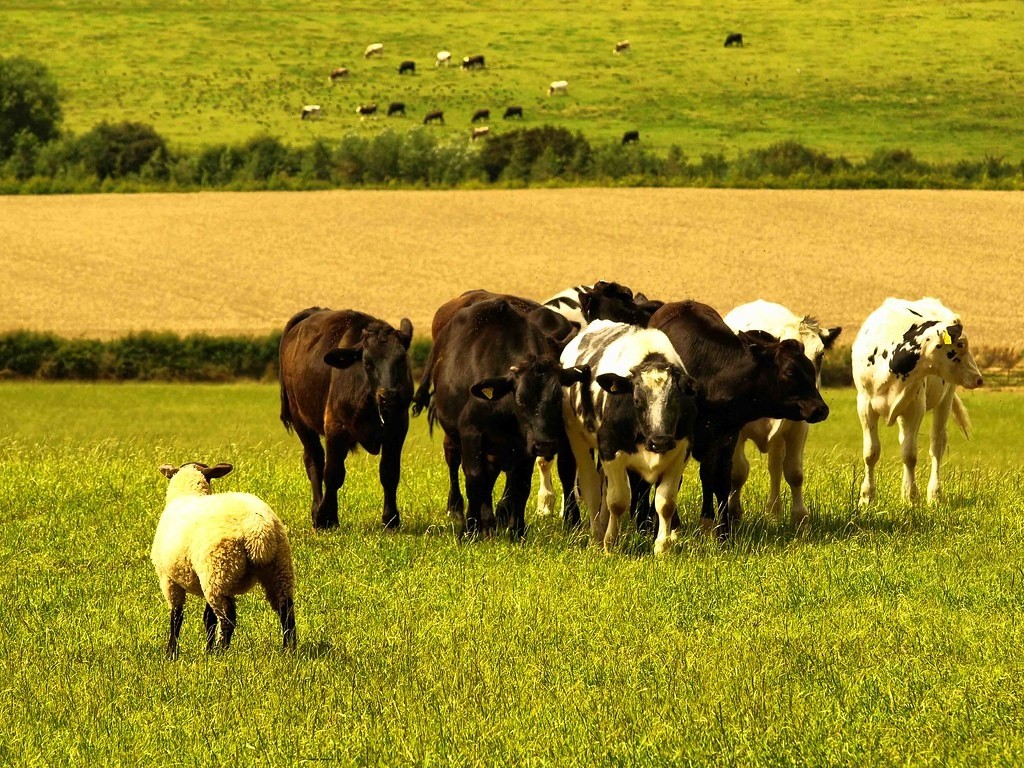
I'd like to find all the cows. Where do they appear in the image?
[851,295,983,514]
[272,284,843,562]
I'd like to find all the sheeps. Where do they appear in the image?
[147,461,299,655]
